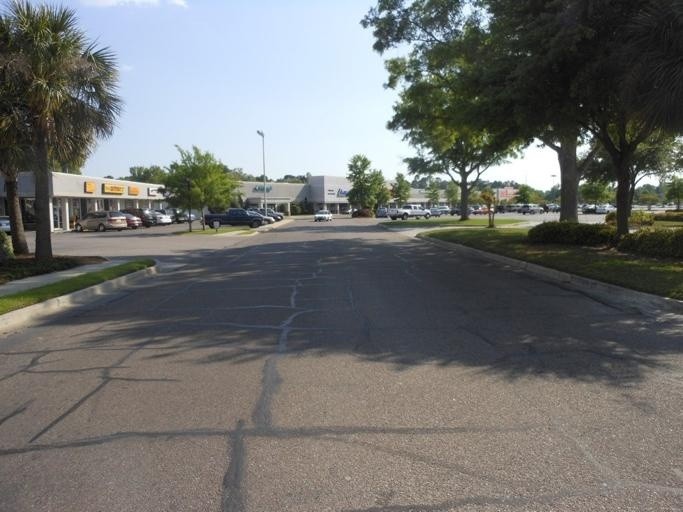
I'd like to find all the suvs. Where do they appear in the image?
[505,203,561,215]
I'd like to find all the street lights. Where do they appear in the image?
[257,131,267,217]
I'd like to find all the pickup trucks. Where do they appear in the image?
[389,204,432,220]
[204,208,284,229]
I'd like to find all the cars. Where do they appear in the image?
[314,209,332,222]
[576,203,618,214]
[428,205,495,217]
[74,208,195,232]
[0,216,11,235]
[376,207,388,217]
